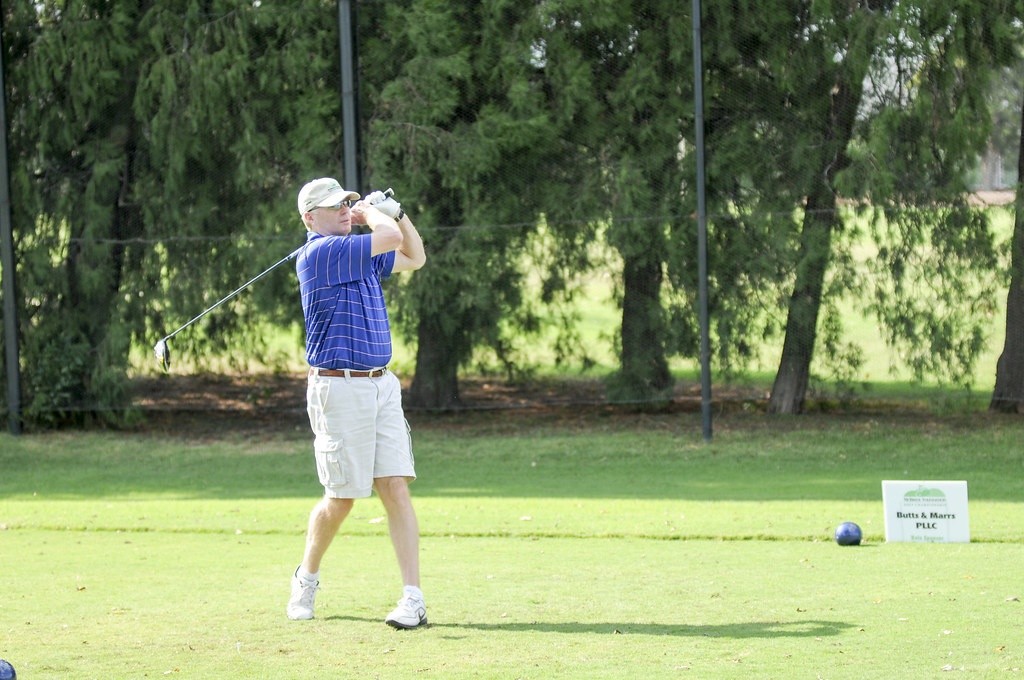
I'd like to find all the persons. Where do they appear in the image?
[286,176,428,629]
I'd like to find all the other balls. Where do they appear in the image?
[835,522,861,546]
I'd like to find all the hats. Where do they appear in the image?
[298,177,361,215]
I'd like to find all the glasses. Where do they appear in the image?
[309,198,354,210]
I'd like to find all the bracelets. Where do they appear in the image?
[394,209,404,222]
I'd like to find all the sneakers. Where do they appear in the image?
[286,566,320,620]
[384,592,430,628]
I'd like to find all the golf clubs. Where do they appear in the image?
[154,187,396,375]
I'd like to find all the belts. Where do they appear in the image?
[309,366,390,378]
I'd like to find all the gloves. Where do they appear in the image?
[364,191,401,217]
